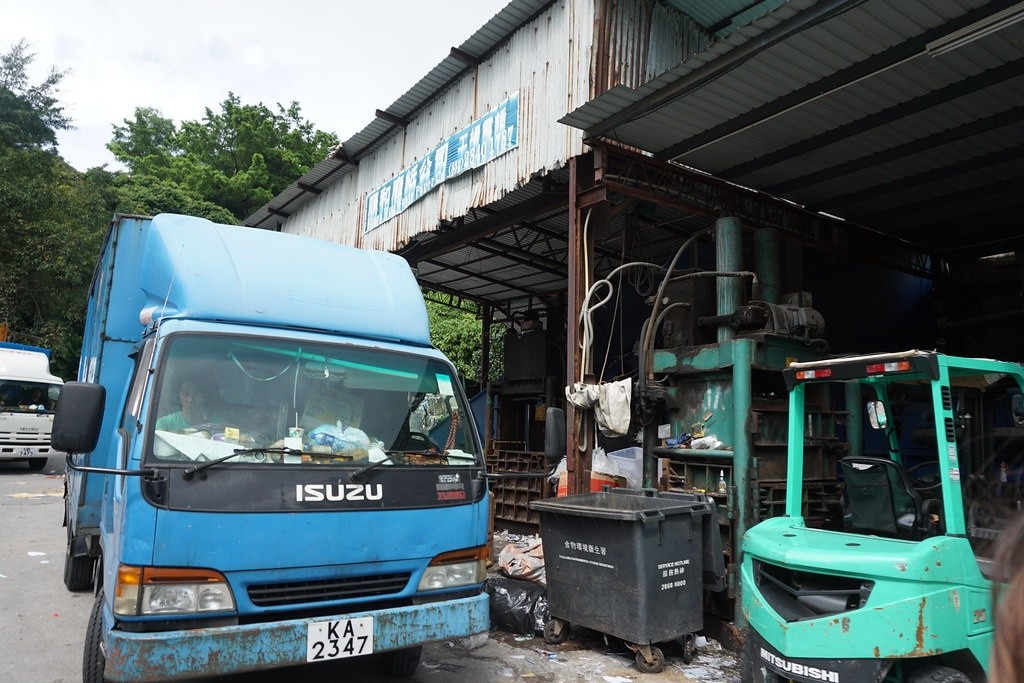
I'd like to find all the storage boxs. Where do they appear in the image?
[557,470,627,499]
[608,445,664,480]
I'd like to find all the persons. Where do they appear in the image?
[156,376,231,434]
[17,388,52,411]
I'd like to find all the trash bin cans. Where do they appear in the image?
[529,484,726,673]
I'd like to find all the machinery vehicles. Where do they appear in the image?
[736,349,1024,683]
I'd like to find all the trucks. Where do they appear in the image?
[49,211,565,683]
[1,341,65,472]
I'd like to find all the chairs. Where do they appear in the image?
[836,455,923,537]
